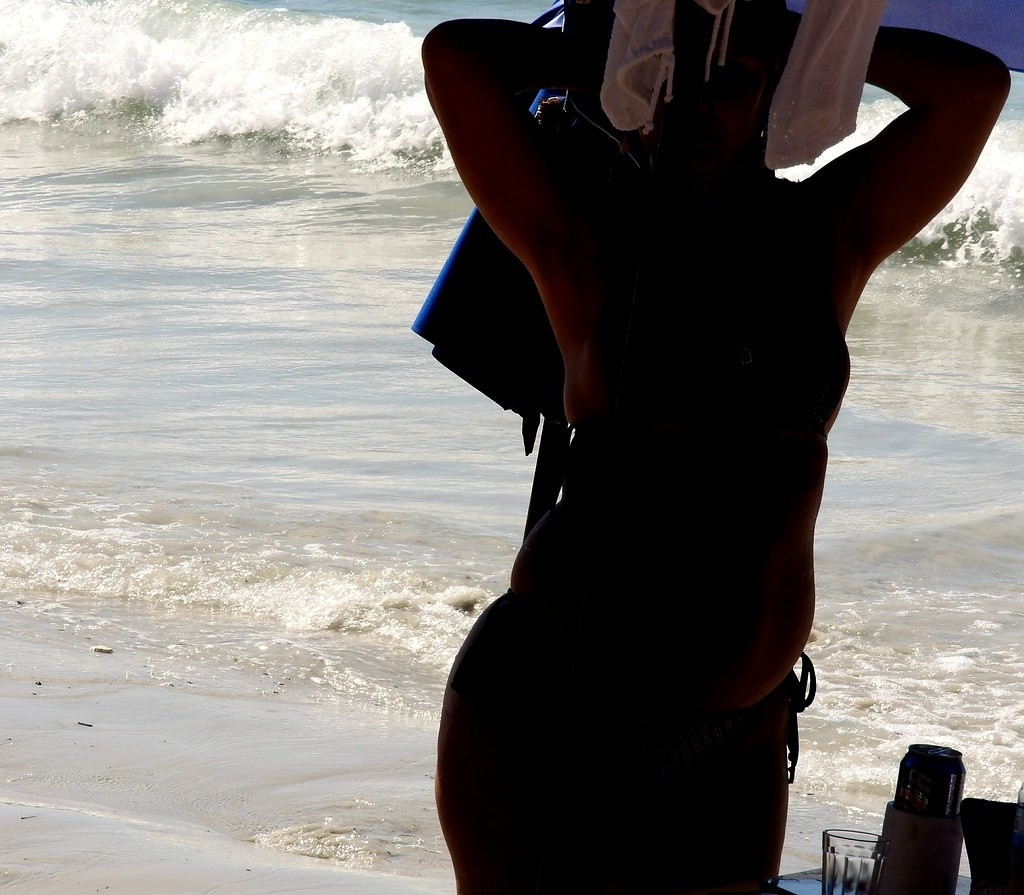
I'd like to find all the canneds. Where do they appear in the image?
[893,744,966,817]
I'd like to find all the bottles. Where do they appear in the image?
[1012,780,1024,848]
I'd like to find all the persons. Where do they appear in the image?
[422,0,1013,895]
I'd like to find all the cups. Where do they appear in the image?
[821,828,890,895]
[870,801,963,895]
